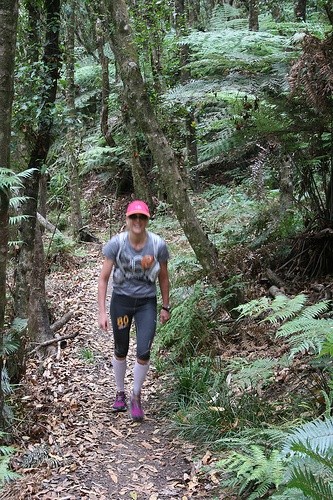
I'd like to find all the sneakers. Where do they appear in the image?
[112,391,127,412]
[130,396,144,421]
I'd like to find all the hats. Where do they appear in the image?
[126,201,150,218]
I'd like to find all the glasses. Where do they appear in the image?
[128,214,147,220]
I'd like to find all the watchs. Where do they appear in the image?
[162,305,170,313]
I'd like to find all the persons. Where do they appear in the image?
[98,201,172,420]
